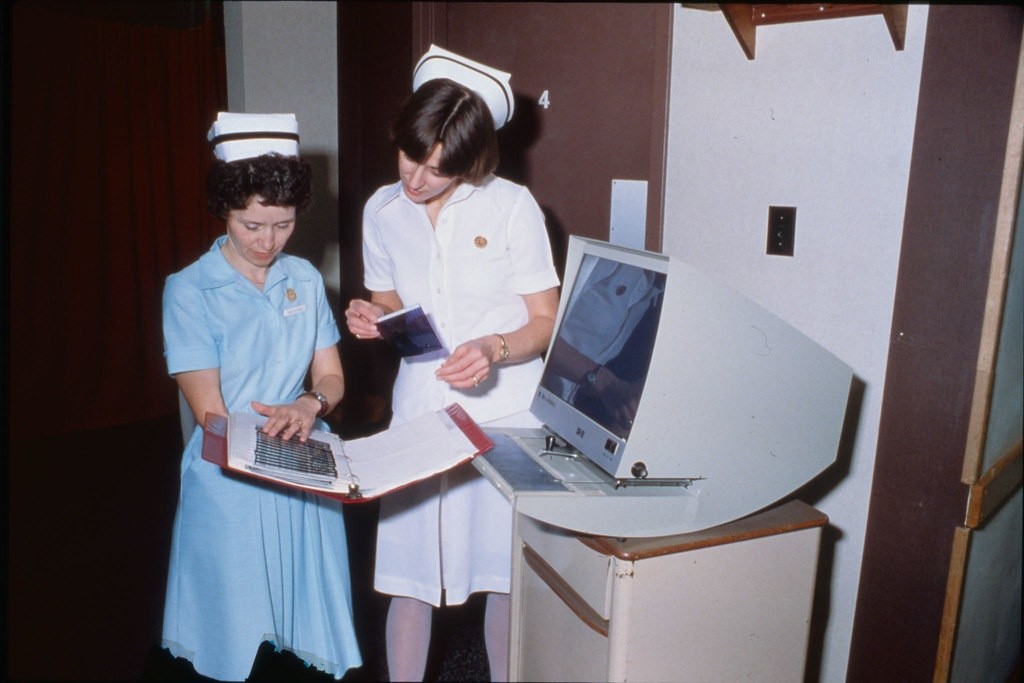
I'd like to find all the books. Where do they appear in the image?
[200,401,497,506]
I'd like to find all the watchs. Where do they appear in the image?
[295,389,329,418]
[492,330,512,370]
[585,364,601,392]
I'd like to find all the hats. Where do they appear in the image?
[206,110,299,162]
[413,43,515,131]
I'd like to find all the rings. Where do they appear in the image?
[356,334,361,340]
[297,420,303,427]
[358,313,363,318]
[473,376,480,388]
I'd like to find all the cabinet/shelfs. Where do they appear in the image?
[505,496,829,683]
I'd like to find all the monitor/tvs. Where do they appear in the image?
[539,253,666,443]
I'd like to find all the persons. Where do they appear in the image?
[344,76,561,683]
[159,150,364,683]
[542,254,665,429]
